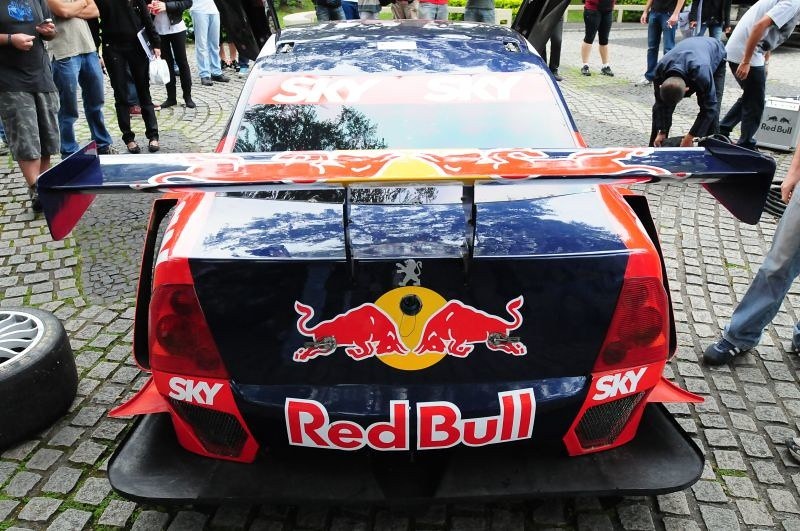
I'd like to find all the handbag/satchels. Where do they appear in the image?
[149,55,171,85]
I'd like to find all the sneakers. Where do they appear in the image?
[0,142,10,154]
[581,65,591,76]
[784,438,800,462]
[703,339,749,365]
[97,145,119,155]
[130,106,143,116]
[154,104,161,110]
[791,339,800,359]
[601,66,614,76]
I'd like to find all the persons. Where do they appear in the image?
[689,0,731,43]
[464,0,495,23]
[581,0,615,77]
[146,0,196,109]
[636,0,685,86]
[390,0,420,19]
[719,0,800,152]
[0,0,118,212]
[419,0,449,20]
[312,0,382,22]
[86,0,162,154]
[648,36,727,148]
[540,15,564,81]
[702,141,800,365]
[188,0,271,86]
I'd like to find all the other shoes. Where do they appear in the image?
[201,78,214,86]
[127,143,141,153]
[183,94,196,108]
[146,140,161,152]
[211,75,229,82]
[220,60,232,70]
[160,99,177,107]
[635,80,652,87]
[239,66,249,77]
[28,184,39,200]
[554,75,563,81]
[231,60,240,69]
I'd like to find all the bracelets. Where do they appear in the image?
[8,34,12,48]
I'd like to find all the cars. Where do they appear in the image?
[27,20,775,516]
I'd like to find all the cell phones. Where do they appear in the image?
[38,19,52,26]
